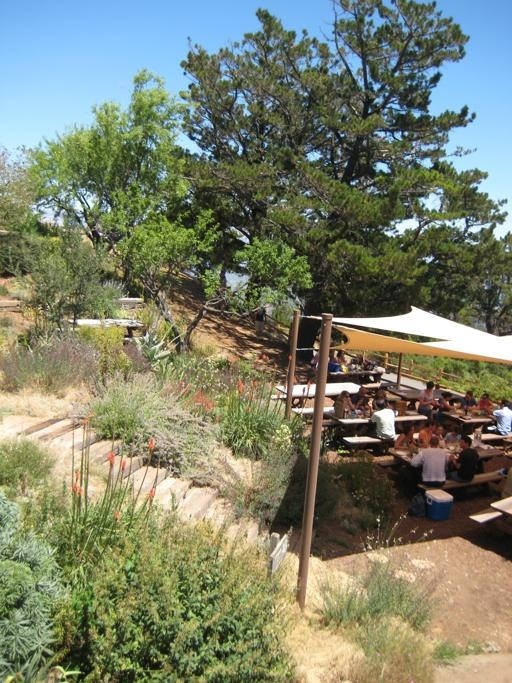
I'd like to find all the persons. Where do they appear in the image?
[254,307,267,338]
[308,339,511,499]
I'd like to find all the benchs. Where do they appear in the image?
[271,369,512,537]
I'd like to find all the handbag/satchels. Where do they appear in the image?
[408,493,426,518]
